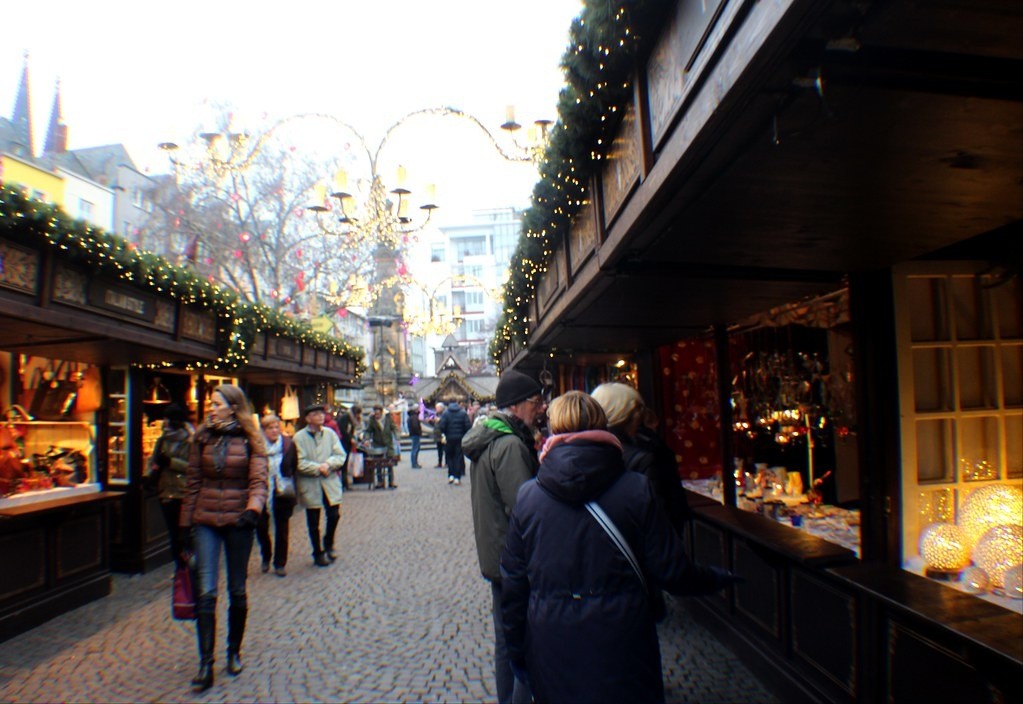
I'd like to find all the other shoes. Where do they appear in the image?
[312,554,328,566]
[323,549,336,559]
[448,475,454,482]
[453,478,459,485]
[375,482,384,488]
[411,464,421,468]
[389,482,397,487]
[274,568,287,575]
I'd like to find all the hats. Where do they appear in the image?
[304,405,323,416]
[408,402,419,410]
[495,370,541,408]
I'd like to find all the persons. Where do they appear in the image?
[149,402,197,575]
[245,414,299,576]
[407,403,423,469]
[462,371,541,704]
[530,382,669,622]
[323,404,402,493]
[280,405,347,567]
[425,396,496,490]
[181,384,268,686]
[500,391,672,704]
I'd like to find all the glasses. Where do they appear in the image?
[525,399,540,407]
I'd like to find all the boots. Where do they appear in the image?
[227,592,246,673]
[192,598,216,685]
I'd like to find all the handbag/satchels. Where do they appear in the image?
[346,446,363,477]
[172,560,198,620]
[275,476,295,498]
